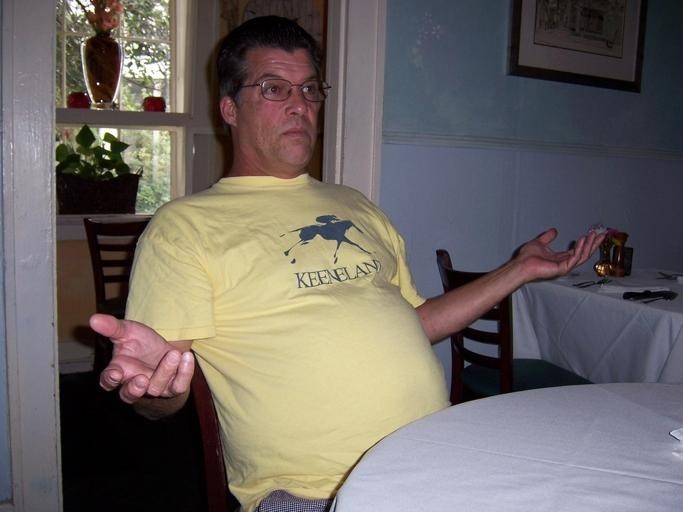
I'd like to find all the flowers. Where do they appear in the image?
[79,1,125,31]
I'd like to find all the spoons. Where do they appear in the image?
[643,292,678,303]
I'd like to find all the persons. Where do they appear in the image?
[90,16,608,512]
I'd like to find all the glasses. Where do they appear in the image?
[239,79,331,102]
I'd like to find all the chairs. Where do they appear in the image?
[190,344,252,511]
[435,248,594,410]
[81,215,151,378]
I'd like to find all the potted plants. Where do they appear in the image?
[53,124,143,215]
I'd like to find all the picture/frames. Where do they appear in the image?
[506,0,650,94]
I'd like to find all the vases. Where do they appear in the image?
[80,27,121,105]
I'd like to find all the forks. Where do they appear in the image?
[659,271,683,277]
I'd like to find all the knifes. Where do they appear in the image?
[579,280,613,288]
[656,277,677,280]
